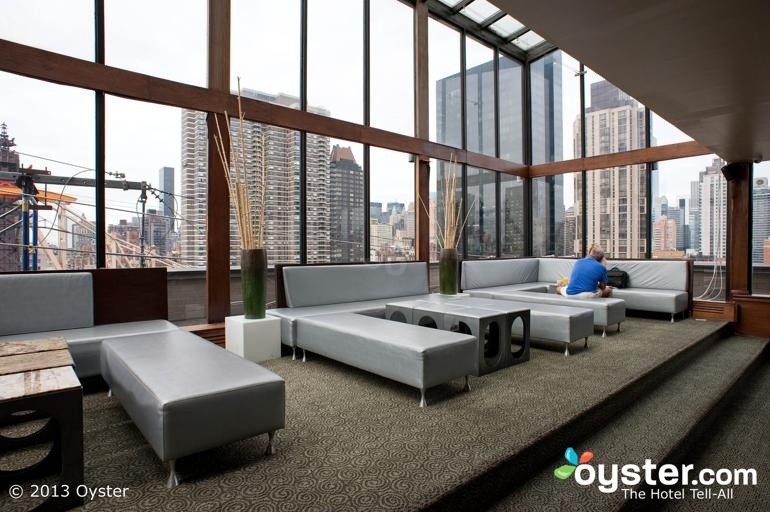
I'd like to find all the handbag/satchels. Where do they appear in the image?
[606,266,628,289]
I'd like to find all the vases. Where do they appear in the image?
[240,247,268,320]
[438,249,458,294]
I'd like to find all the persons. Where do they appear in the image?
[588,243,608,265]
[556,248,608,299]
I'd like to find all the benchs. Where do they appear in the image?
[0,267,177,381]
[450,297,594,357]
[267,262,432,361]
[99,325,286,492]
[460,254,694,324]
[495,291,626,338]
[299,313,478,409]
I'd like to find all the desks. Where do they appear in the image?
[385,297,530,377]
[434,292,470,298]
[224,314,282,363]
[1,334,88,512]
